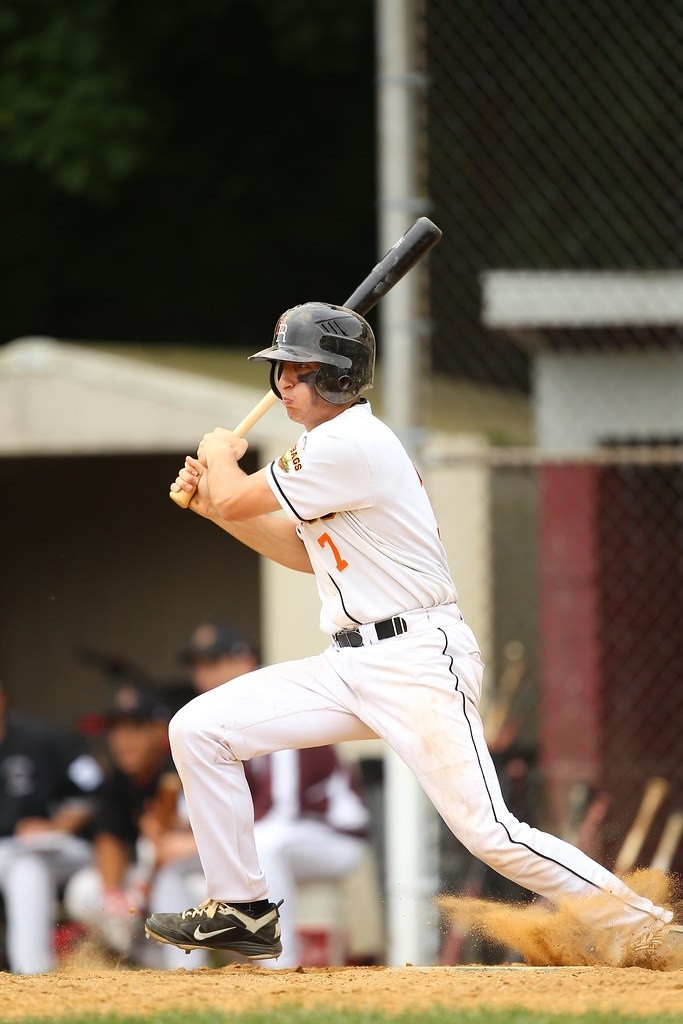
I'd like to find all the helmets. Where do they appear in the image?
[247,299,378,405]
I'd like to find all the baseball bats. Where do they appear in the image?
[166,216,444,511]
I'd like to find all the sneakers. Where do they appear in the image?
[616,903,683,969]
[141,896,286,959]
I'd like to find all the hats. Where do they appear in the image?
[176,620,257,667]
[93,675,157,723]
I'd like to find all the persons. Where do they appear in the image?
[145,303,675,970]
[0,628,371,977]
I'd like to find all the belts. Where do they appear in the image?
[332,616,408,648]
